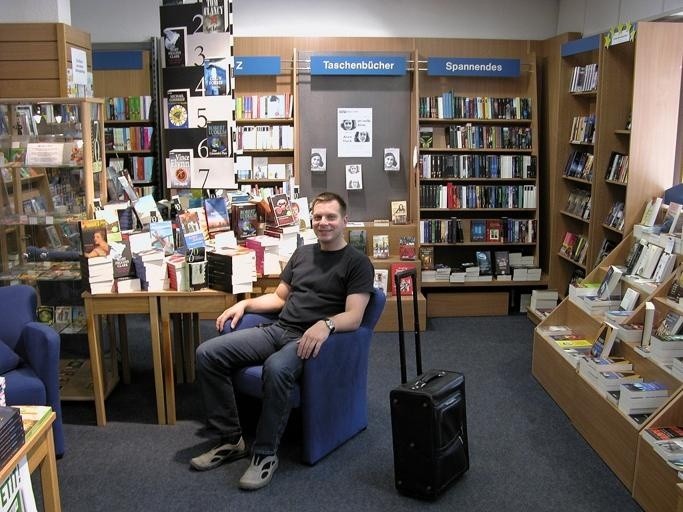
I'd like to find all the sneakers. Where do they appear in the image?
[191,437,247,471]
[240,453,279,489]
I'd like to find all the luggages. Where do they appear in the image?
[390,268,470,502]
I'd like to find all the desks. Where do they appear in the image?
[81,272,282,425]
[0,411,62,512]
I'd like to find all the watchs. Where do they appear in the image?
[321,316,335,336]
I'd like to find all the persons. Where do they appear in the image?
[188,190,376,491]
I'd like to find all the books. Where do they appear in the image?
[320,64,629,311]
[0,390,55,512]
[532,196,683,489]
[158,1,238,96]
[1,95,320,390]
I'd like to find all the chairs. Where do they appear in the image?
[219,287,386,465]
[0,284,66,457]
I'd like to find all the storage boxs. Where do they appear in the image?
[427,288,509,317]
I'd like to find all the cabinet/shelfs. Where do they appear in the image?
[548,33,604,301]
[0,98,120,403]
[588,21,683,272]
[417,36,548,287]
[92,50,158,202]
[294,37,427,333]
[158,37,295,201]
